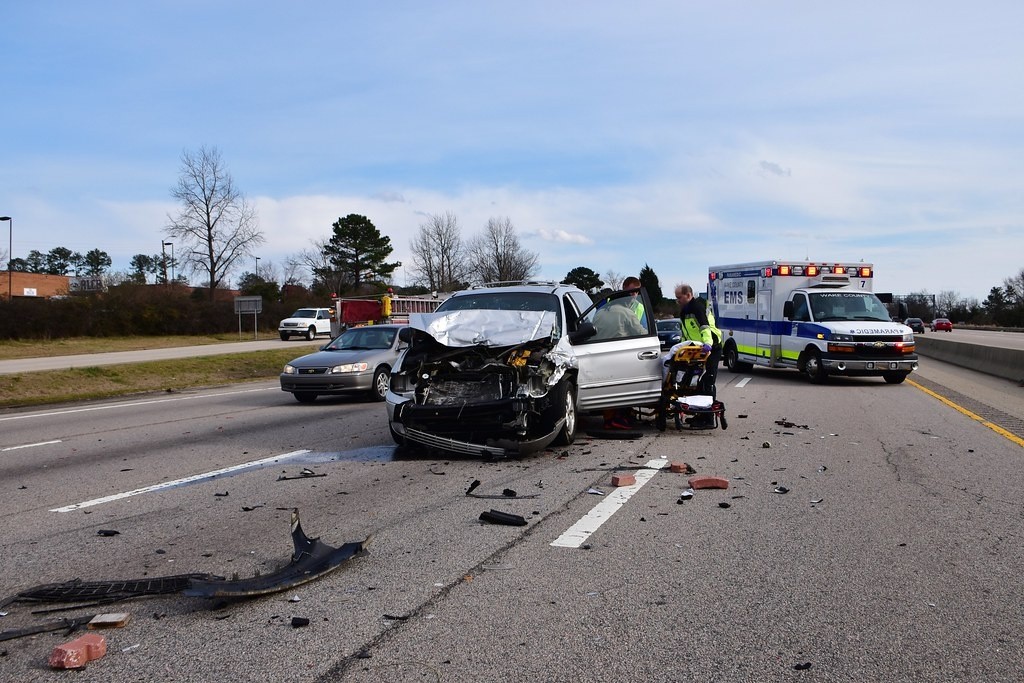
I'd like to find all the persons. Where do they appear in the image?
[594,275,658,337]
[674,284,723,429]
[590,293,652,431]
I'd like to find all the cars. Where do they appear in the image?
[904,318,925,334]
[277,307,330,341]
[385,279,665,460]
[656,318,683,346]
[279,324,409,403]
[930,318,952,332]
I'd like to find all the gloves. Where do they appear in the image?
[702,343,712,351]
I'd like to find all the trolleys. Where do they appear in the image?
[631,345,724,430]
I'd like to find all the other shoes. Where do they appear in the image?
[685,415,714,428]
[629,408,643,424]
[603,412,636,430]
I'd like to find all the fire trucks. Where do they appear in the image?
[330,291,501,348]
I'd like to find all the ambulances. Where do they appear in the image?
[706,260,919,384]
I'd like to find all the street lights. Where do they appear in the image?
[256,257,261,276]
[0,216,13,302]
[164,242,174,289]
